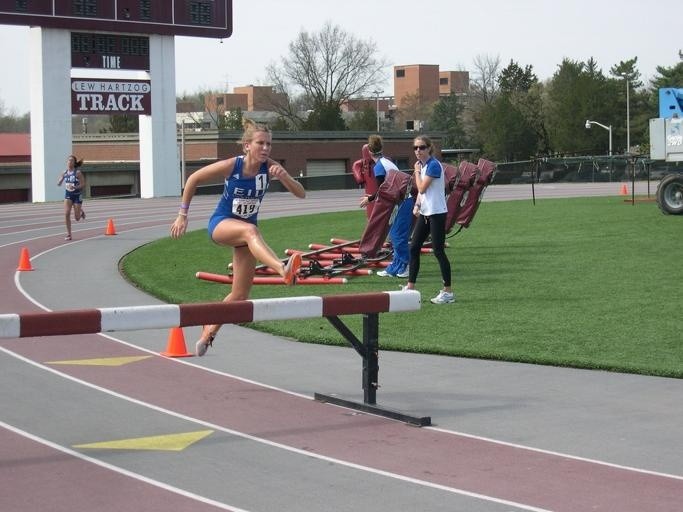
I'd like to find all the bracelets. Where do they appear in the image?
[178,202,190,219]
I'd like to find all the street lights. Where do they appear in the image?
[621,72,633,152]
[584,119,614,156]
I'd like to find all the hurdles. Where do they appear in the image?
[0,289,433,427]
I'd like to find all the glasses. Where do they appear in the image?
[414,145,430,150]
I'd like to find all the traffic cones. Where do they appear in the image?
[104,218,118,235]
[160,328,194,358]
[620,184,630,195]
[16,247,35,271]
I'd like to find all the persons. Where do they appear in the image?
[359,134,455,304]
[170,117,306,358]
[56,155,86,241]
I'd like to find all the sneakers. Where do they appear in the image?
[430,290,455,304]
[196,323,216,357]
[81,209,86,220]
[396,265,409,277]
[376,270,394,277]
[65,235,72,241]
[282,253,302,285]
[402,285,412,290]
